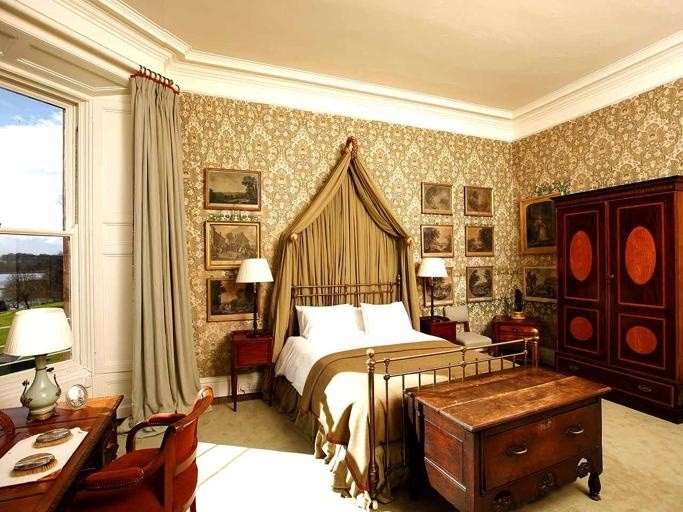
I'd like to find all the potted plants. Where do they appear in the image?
[511,288,525,320]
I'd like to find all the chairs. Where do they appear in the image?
[444,304,492,348]
[65,384,214,512]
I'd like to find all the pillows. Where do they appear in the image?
[293,300,412,339]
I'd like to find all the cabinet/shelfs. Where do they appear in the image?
[491,315,540,365]
[405,362,611,512]
[548,174,681,423]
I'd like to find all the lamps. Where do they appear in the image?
[416,257,448,322]
[3,308,72,425]
[235,258,275,338]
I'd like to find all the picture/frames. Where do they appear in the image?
[204,167,262,212]
[464,265,495,303]
[464,225,494,257]
[522,264,557,304]
[419,222,455,258]
[463,185,494,217]
[517,191,562,255]
[64,383,88,411]
[205,278,261,322]
[420,180,453,216]
[203,220,261,271]
[423,266,454,308]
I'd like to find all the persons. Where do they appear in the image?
[532,213,552,242]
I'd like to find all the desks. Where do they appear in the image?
[0,394,127,510]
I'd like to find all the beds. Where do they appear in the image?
[277,273,540,512]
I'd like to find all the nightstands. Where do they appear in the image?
[419,313,457,344]
[229,329,276,411]
